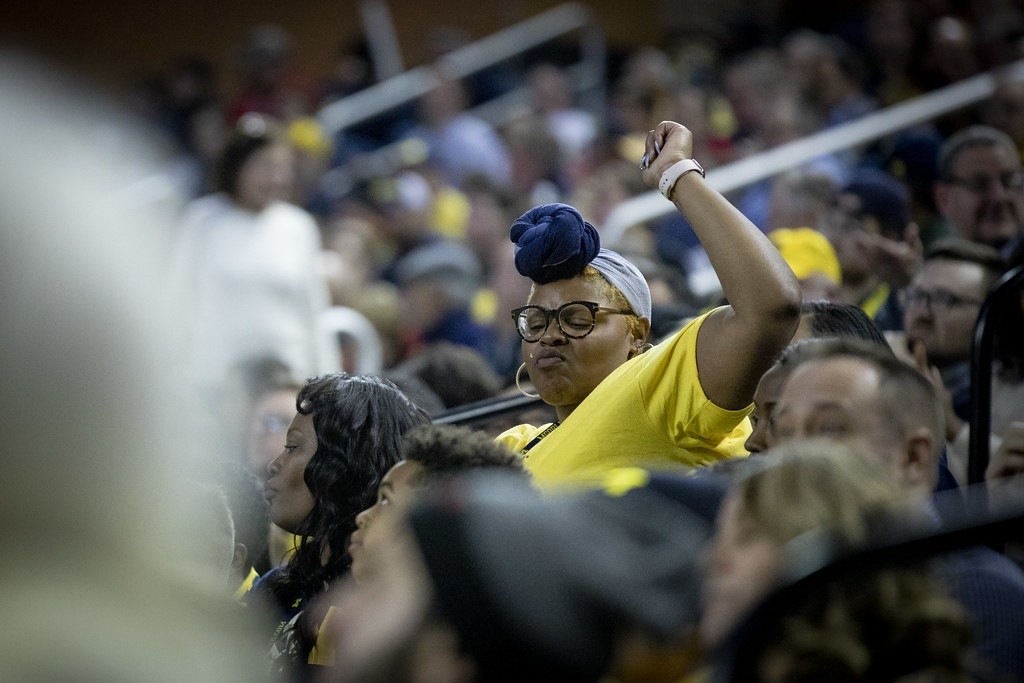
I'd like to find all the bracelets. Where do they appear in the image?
[657,156,705,201]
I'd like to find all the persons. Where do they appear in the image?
[0,0,1024,683]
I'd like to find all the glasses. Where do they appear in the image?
[949,171,1024,193]
[901,285,980,314]
[509,299,635,342]
